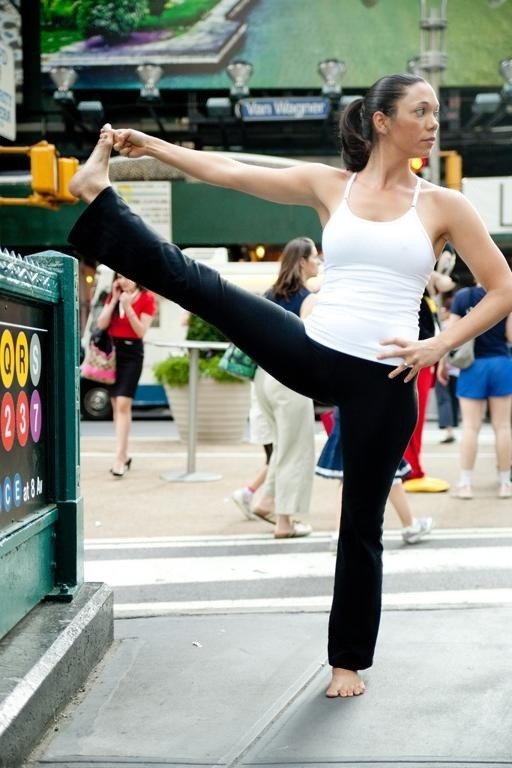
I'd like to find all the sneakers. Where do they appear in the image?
[448,483,473,498]
[402,517,432,541]
[496,481,512,497]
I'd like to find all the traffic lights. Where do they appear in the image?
[33,141,81,207]
[410,154,429,180]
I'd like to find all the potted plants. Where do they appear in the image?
[150,314,257,448]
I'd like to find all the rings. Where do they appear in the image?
[399,359,415,366]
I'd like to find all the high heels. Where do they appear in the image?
[110,457,131,476]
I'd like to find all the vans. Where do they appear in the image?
[79,260,323,421]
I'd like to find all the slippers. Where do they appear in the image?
[230,488,311,539]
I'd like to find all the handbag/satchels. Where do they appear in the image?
[81,334,118,386]
[447,338,476,371]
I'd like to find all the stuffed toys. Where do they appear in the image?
[401,242,457,492]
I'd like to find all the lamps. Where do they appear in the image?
[492,57,512,102]
[315,54,351,109]
[224,56,255,113]
[127,60,170,137]
[48,59,86,138]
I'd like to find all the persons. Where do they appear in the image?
[64,69,511,700]
[434,277,511,499]
[316,404,434,543]
[231,237,322,538]
[98,266,156,476]
